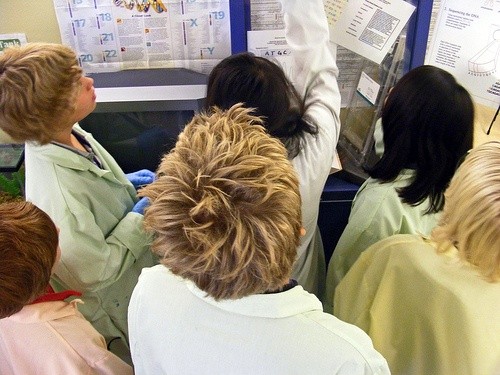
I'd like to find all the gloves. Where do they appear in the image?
[132,197,150,214]
[123,169,156,186]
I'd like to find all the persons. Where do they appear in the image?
[0,42,157,350]
[202,0,342,295]
[320,66,475,308]
[332,139,500,375]
[126,105,390,375]
[0,194,135,374]
